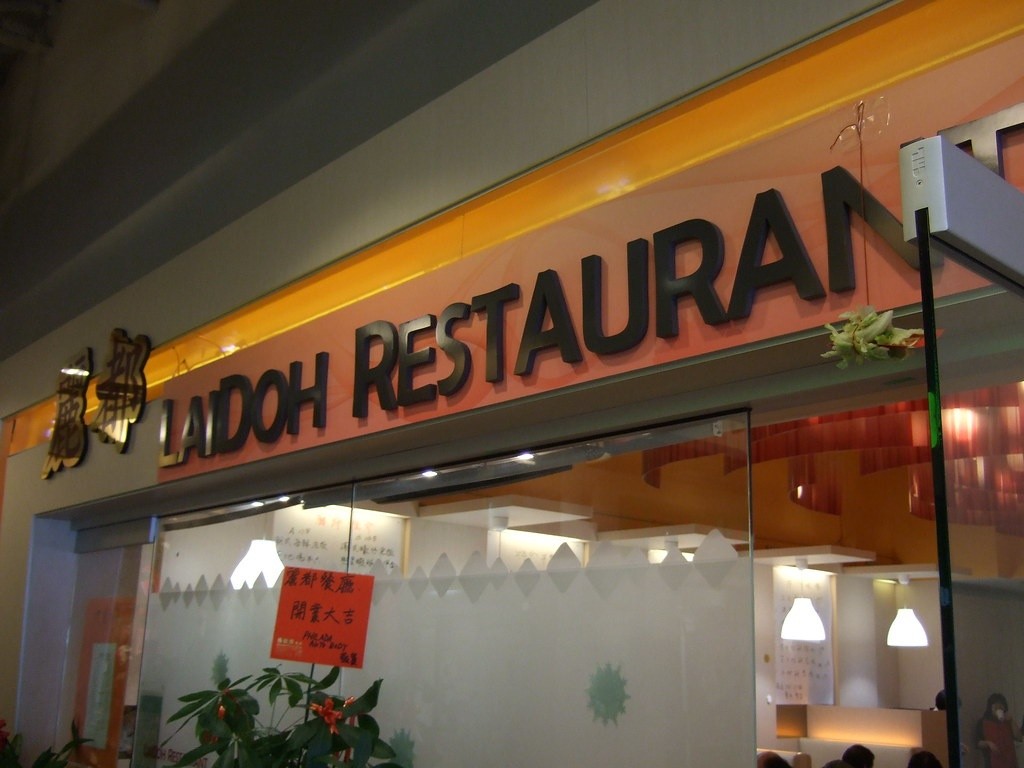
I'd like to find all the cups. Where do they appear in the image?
[996,709,1004,720]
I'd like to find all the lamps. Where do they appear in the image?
[228,511,290,590]
[885,574,928,647]
[780,556,826,642]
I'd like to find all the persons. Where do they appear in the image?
[972,692,1024,768]
[757,744,941,768]
[929,690,970,757]
